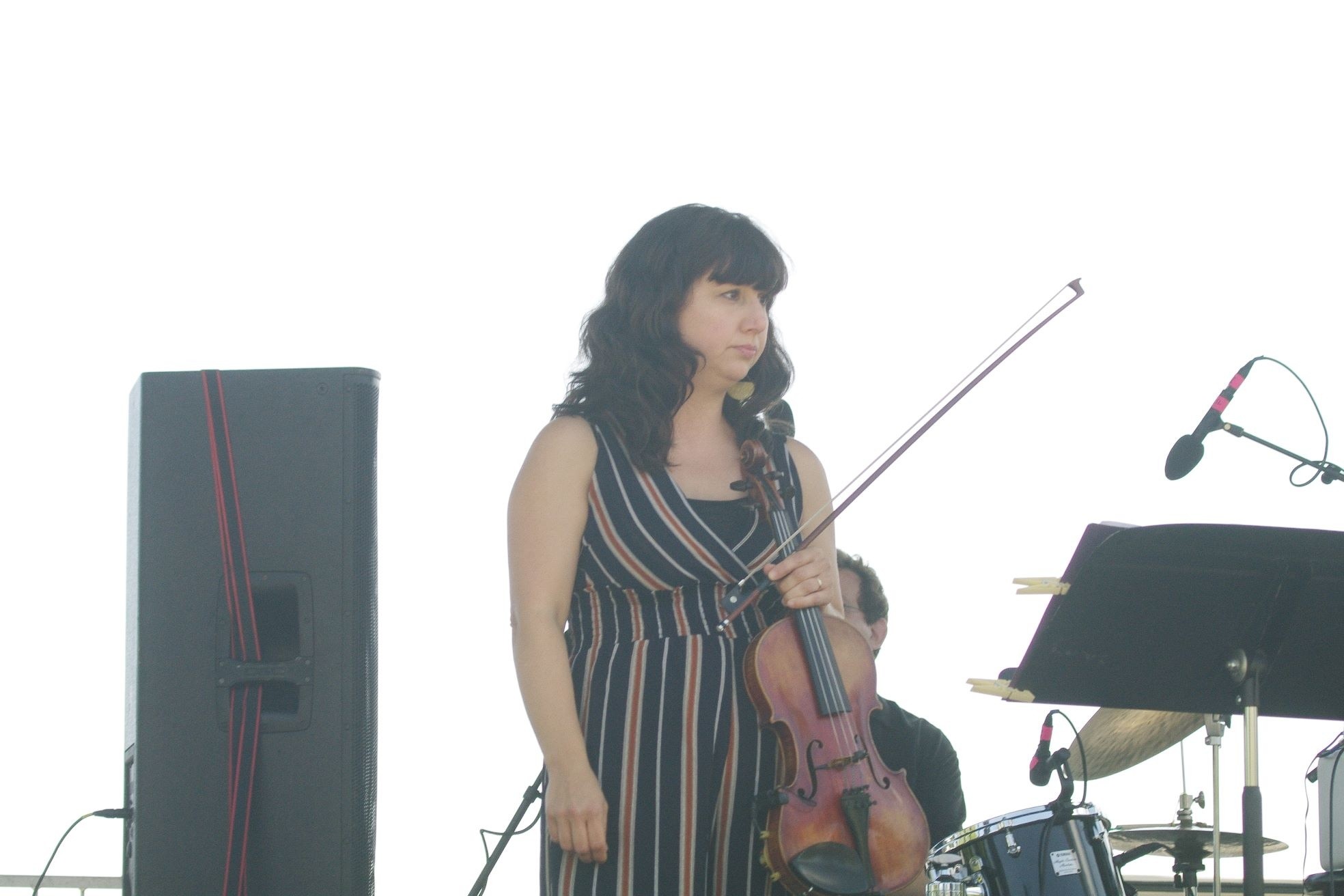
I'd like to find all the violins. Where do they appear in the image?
[727,440,930,895]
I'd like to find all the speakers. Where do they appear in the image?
[1317,742,1344,872]
[118,369,379,896]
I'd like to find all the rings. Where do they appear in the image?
[814,577,823,592]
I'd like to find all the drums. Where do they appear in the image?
[930,798,1127,896]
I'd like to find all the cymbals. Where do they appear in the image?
[1102,823,1292,860]
[1064,708,1230,779]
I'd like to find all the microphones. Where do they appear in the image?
[1030,712,1054,786]
[1165,358,1255,480]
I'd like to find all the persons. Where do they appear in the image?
[835,549,966,896]
[508,203,847,896]
